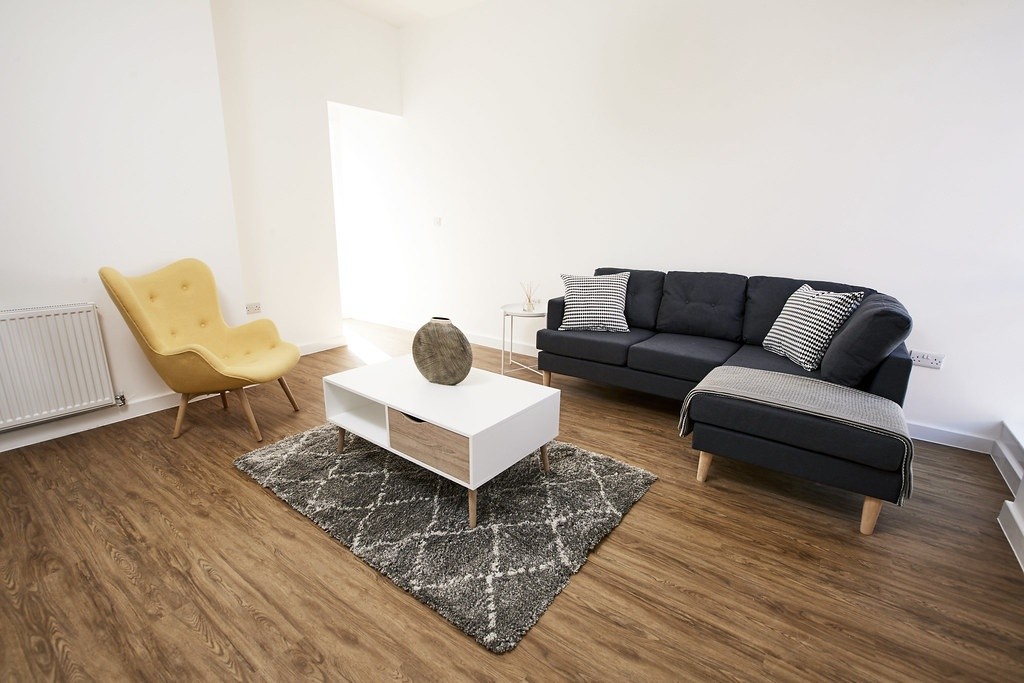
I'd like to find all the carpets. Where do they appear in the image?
[231,422,658,653]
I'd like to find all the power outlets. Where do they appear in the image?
[245,303,262,313]
[910,350,942,369]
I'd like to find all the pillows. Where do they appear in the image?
[558,272,630,332]
[763,284,865,371]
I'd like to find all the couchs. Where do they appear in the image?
[536,268,913,535]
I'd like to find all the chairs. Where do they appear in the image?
[98,258,301,442]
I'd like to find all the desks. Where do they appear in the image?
[500,304,547,375]
[323,354,560,528]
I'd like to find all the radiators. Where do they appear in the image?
[0,302,116,431]
